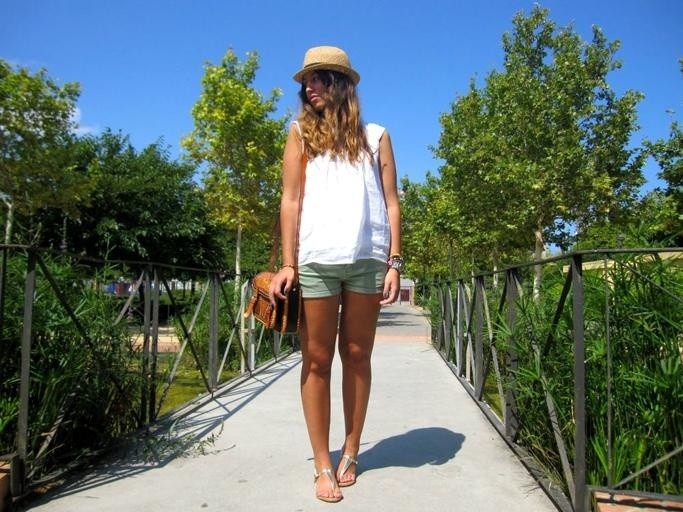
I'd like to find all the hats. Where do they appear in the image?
[292,46,360,86]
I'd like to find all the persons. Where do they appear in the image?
[266,45,403,504]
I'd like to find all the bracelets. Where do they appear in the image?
[386,252,404,271]
[278,265,296,271]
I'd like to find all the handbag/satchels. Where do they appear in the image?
[243,271,301,335]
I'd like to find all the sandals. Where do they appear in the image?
[334,454,358,487]
[312,464,343,503]
